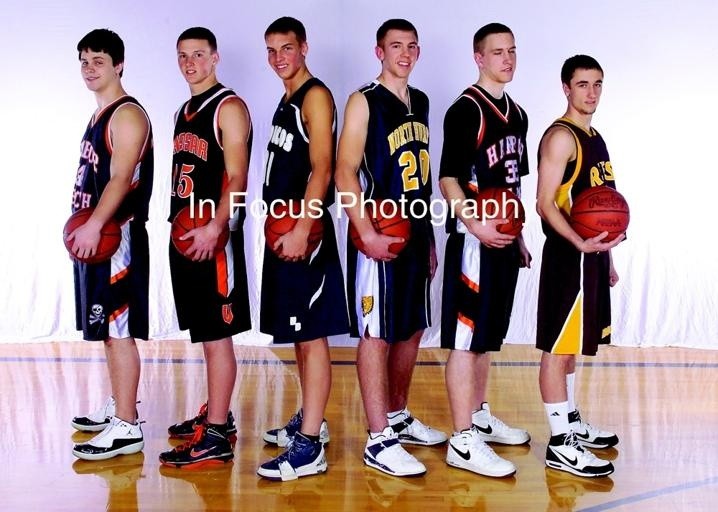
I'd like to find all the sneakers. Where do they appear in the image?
[545,432,614,478]
[263,408,330,447]
[444,427,516,479]
[387,408,447,446]
[159,421,233,470]
[363,427,427,477]
[71,418,144,461]
[168,402,237,443]
[256,432,328,480]
[567,409,619,449]
[71,396,140,433]
[470,401,530,446]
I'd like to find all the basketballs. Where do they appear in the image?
[171,204,230,257]
[64,209,121,263]
[351,202,411,260]
[573,188,628,241]
[474,190,523,244]
[264,204,324,260]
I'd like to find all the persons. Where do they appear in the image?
[65,29,154,461]
[533,55,630,479]
[159,27,253,472]
[333,19,447,478]
[437,23,531,480]
[257,15,349,484]
[69,427,618,512]
